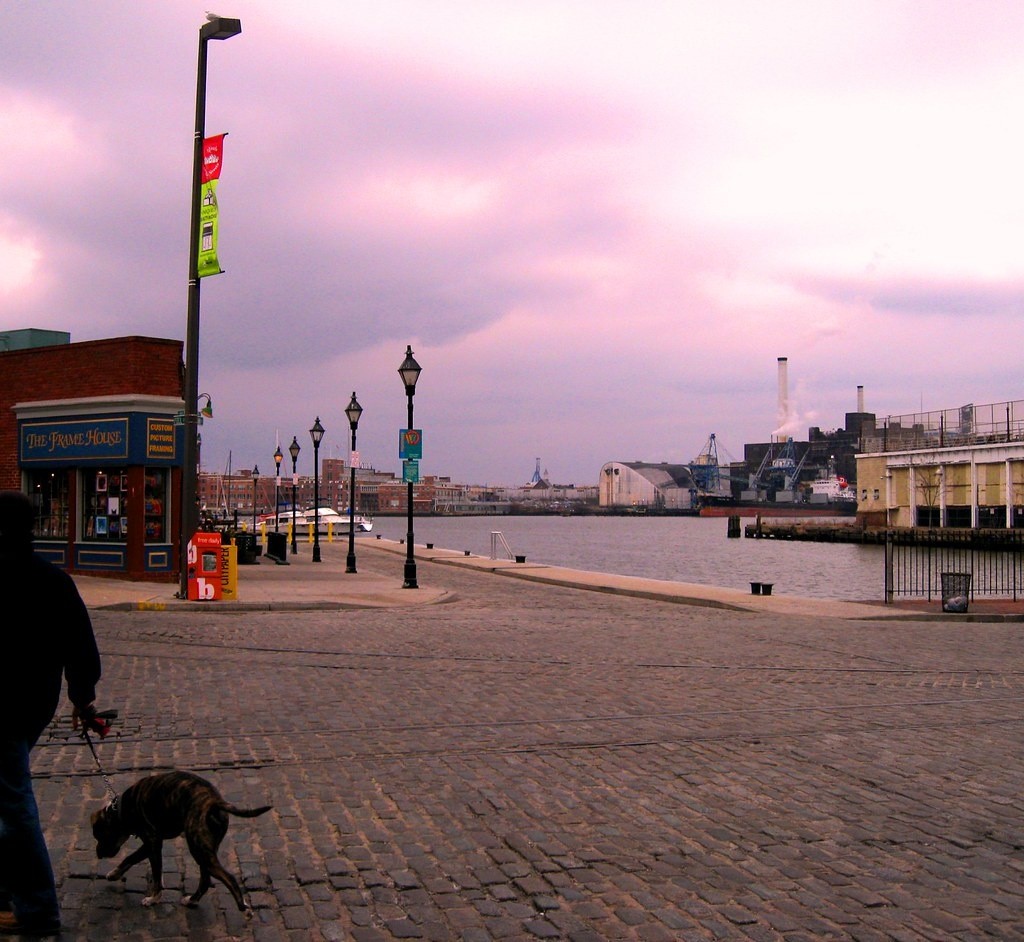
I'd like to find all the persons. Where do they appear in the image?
[0,490,101,936]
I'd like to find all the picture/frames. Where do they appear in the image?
[120,474,128,492]
[120,516,128,534]
[107,497,120,516]
[94,516,108,534]
[95,473,108,493]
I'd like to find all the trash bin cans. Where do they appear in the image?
[187,533,222,601]
[941,573,971,613]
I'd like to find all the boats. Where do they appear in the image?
[249,521,266,533]
[261,506,374,535]
[230,521,247,530]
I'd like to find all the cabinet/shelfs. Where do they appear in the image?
[32,475,69,538]
[146,467,167,543]
[83,471,127,540]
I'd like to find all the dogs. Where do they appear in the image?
[90,771,275,921]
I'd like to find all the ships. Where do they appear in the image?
[696,447,856,517]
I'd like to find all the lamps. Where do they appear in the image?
[198,393,213,419]
[934,468,943,477]
[883,472,892,479]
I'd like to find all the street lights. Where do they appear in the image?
[273,446,283,532]
[396,344,424,588]
[251,464,260,534]
[288,435,301,555]
[343,391,364,573]
[308,416,325,562]
